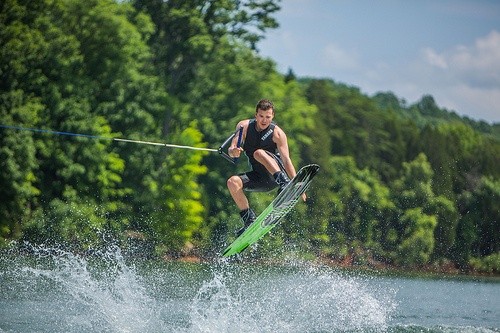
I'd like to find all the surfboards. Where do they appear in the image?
[220,164,321,258]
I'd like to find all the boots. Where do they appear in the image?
[237,208,256,236]
[273,171,291,193]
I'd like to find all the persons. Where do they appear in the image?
[227,99,306,237]
